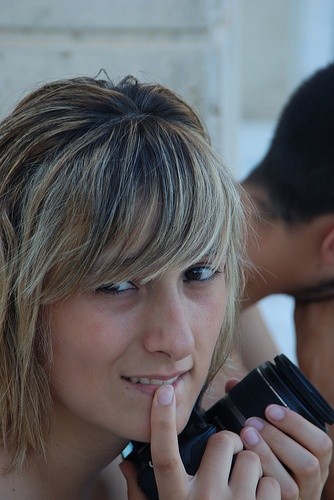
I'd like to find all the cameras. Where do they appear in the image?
[122,354,334,500]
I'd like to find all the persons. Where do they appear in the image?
[0,68,333,500]
[202,62,334,500]
